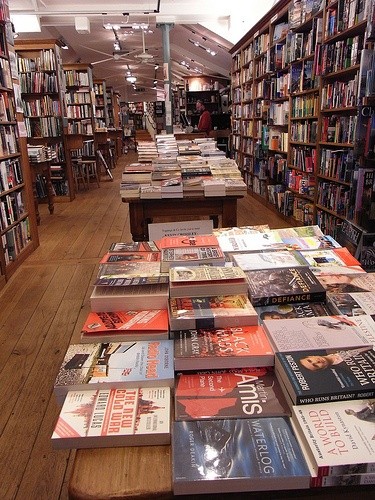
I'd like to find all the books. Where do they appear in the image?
[0,0,375,495]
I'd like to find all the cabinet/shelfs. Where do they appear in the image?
[171,0,375,274]
[0,0,165,293]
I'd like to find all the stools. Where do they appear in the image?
[78,161,100,190]
[71,161,87,193]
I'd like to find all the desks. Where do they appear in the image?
[122,194,244,242]
[30,161,54,225]
[68,444,173,500]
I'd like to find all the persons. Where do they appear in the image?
[345,402,375,421]
[296,346,375,370]
[196,100,212,131]
[258,306,319,320]
[313,275,375,293]
